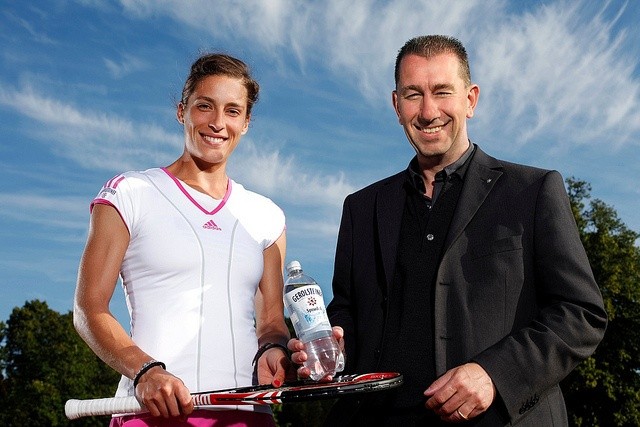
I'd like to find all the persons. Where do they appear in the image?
[282,33,609,426]
[71,50,291,426]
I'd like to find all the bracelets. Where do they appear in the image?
[131,359,166,388]
[252,341,289,370]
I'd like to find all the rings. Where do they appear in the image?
[456,409,470,422]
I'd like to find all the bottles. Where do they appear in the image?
[283,260,345,383]
